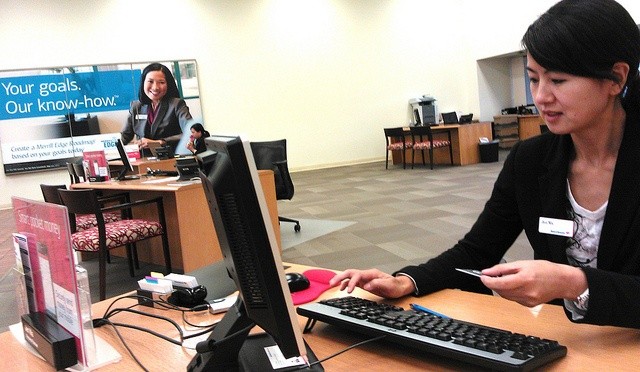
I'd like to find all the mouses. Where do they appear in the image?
[285,272,310,293]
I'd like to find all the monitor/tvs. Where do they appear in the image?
[186,135,325,372]
[114,138,140,182]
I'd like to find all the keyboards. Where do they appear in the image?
[296,296,567,372]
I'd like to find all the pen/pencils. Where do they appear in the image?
[410,303,450,318]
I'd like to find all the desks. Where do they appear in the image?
[391,121,492,165]
[0,260,640,372]
[518,114,547,141]
[72,160,281,274]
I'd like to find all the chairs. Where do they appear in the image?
[410,125,453,170]
[249,139,300,234]
[56,188,171,300]
[40,184,139,275]
[65,162,77,184]
[72,163,85,182]
[384,127,425,169]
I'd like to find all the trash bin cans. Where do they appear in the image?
[477,140,499,163]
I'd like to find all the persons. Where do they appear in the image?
[185,123,210,155]
[120,64,192,156]
[329,0,638,328]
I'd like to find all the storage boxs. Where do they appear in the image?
[495,137,519,148]
[493,115,518,127]
[494,127,518,136]
